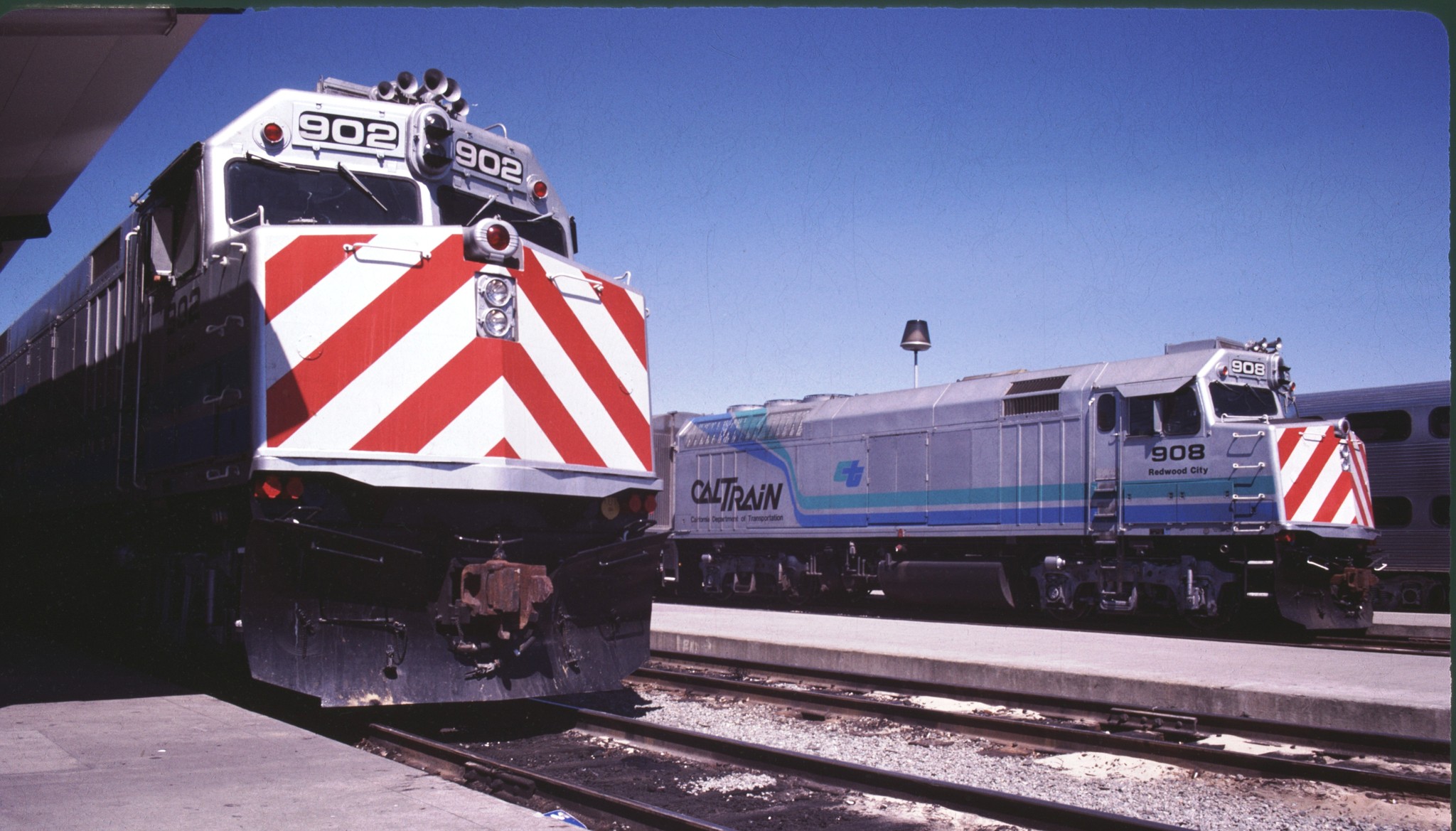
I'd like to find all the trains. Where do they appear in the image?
[652,339,1391,641]
[1283,378,1452,613]
[1,68,667,709]
